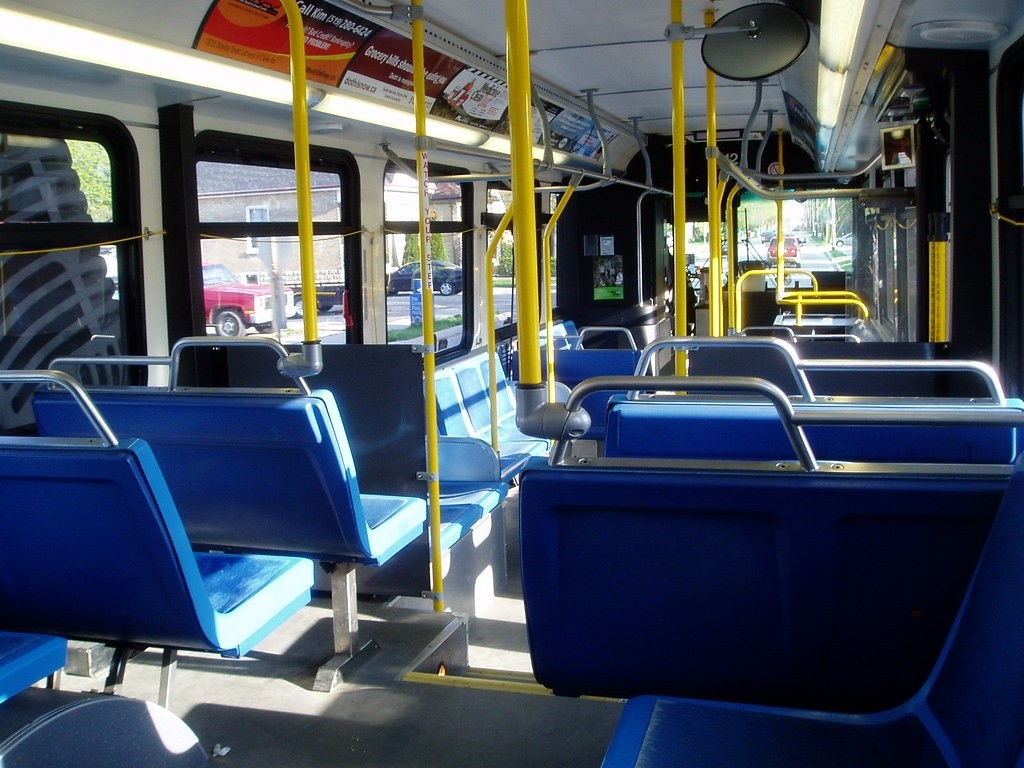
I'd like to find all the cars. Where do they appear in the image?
[767,236,801,269]
[760,230,774,245]
[836,232,853,248]
[385,258,463,296]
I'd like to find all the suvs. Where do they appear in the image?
[202,262,297,338]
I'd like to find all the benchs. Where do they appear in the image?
[0,309,1024,768]
